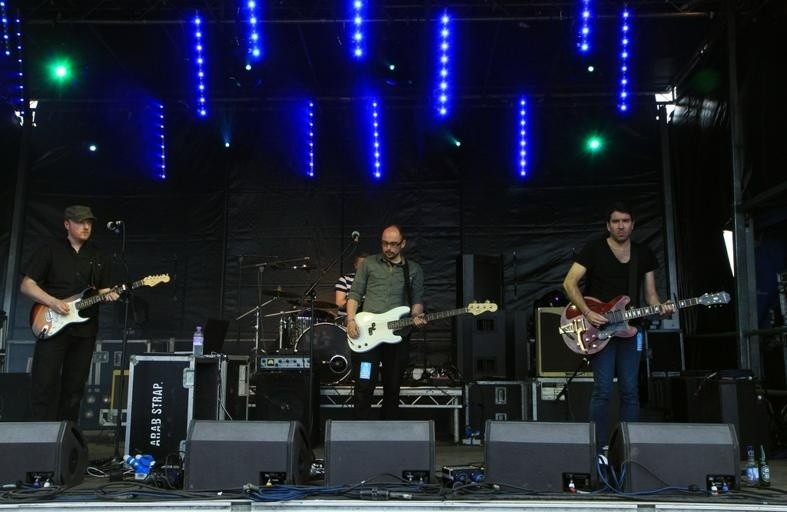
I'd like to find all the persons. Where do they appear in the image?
[335,253,365,327]
[20,205,121,421]
[345,224,425,420]
[558,201,675,453]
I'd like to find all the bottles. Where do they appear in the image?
[192,326,204,356]
[746,444,771,489]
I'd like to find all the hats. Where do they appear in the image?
[64,205,96,222]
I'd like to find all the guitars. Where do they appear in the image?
[30,273,170,341]
[559,290,731,356]
[347,299,499,354]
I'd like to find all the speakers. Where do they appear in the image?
[453,312,529,381]
[456,252,505,311]
[607,421,742,496]
[183,419,314,492]
[0,422,86,490]
[324,419,435,493]
[484,419,598,493]
[255,353,320,440]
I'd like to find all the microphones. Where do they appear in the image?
[352,231,360,246]
[106,220,121,231]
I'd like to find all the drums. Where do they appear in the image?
[273,315,328,349]
[302,306,337,323]
[294,322,355,386]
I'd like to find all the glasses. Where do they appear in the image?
[383,240,403,247]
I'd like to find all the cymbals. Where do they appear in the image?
[259,284,298,299]
[287,299,338,307]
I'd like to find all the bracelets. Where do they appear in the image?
[583,309,591,317]
[346,318,354,324]
